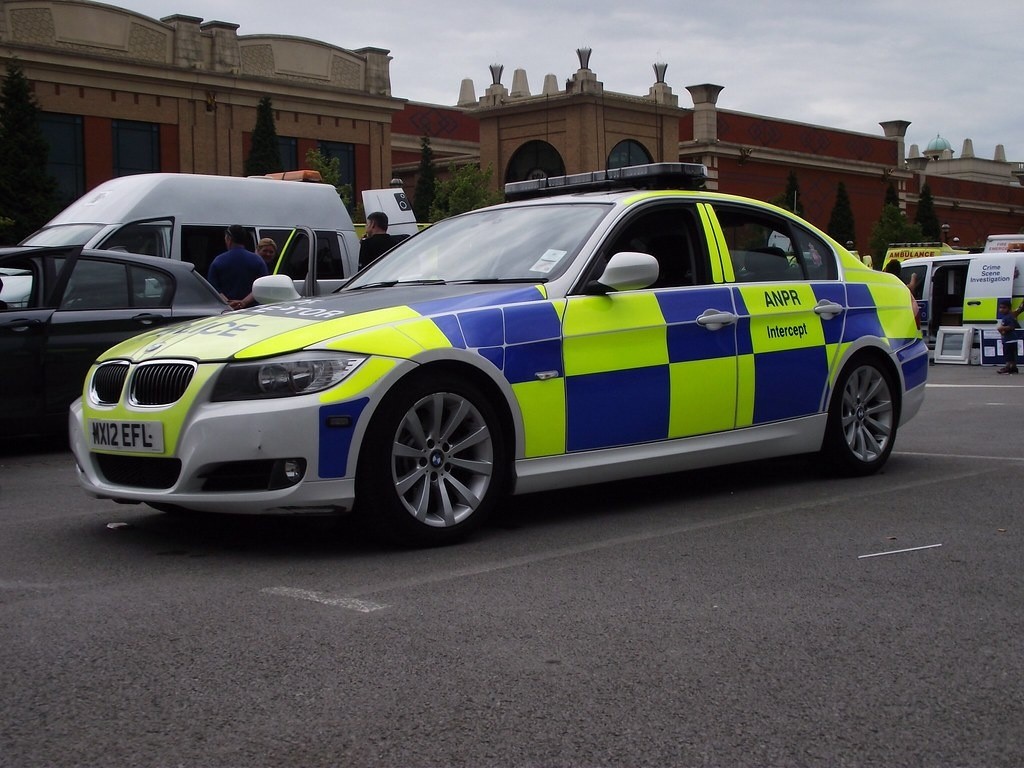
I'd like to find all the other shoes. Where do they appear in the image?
[998,365,1018,373]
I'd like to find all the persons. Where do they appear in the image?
[358,212,397,273]
[257,238,277,268]
[207,225,265,310]
[883,259,917,291]
[997,300,1024,374]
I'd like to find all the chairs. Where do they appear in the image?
[745,247,790,282]
[641,223,705,286]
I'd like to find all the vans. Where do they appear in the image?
[0,171,421,309]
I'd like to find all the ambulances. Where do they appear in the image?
[882,234,1024,351]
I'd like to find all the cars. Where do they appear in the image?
[69,162,931,552]
[1,242,236,448]
